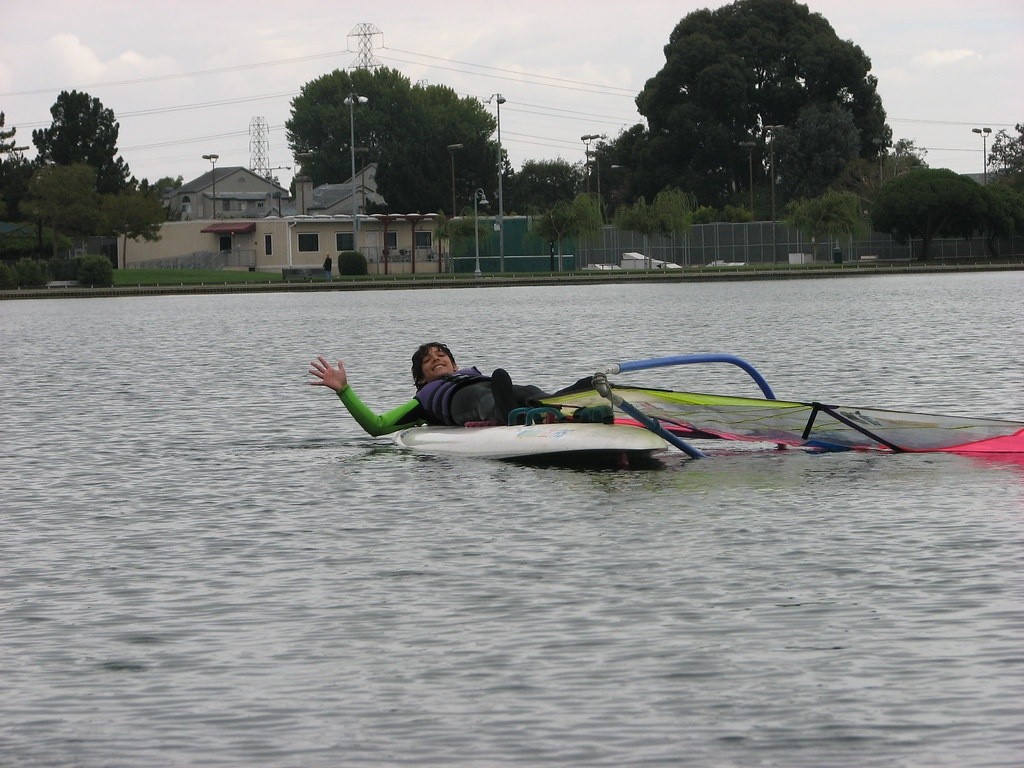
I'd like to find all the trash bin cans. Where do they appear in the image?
[834,253,842,264]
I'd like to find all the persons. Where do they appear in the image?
[323,252,334,283]
[309,341,597,435]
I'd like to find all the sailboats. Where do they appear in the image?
[392,353,1023,466]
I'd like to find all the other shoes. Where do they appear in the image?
[491,368,522,426]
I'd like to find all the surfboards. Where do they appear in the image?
[390,419,669,457]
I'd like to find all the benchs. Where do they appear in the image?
[282,268,327,283]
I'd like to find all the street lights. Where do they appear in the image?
[202,154,218,219]
[252,166,291,215]
[766,125,783,263]
[973,128,992,187]
[582,134,599,193]
[344,92,368,251]
[448,143,462,219]
[483,93,506,273]
[474,187,489,277]
[738,141,756,215]
